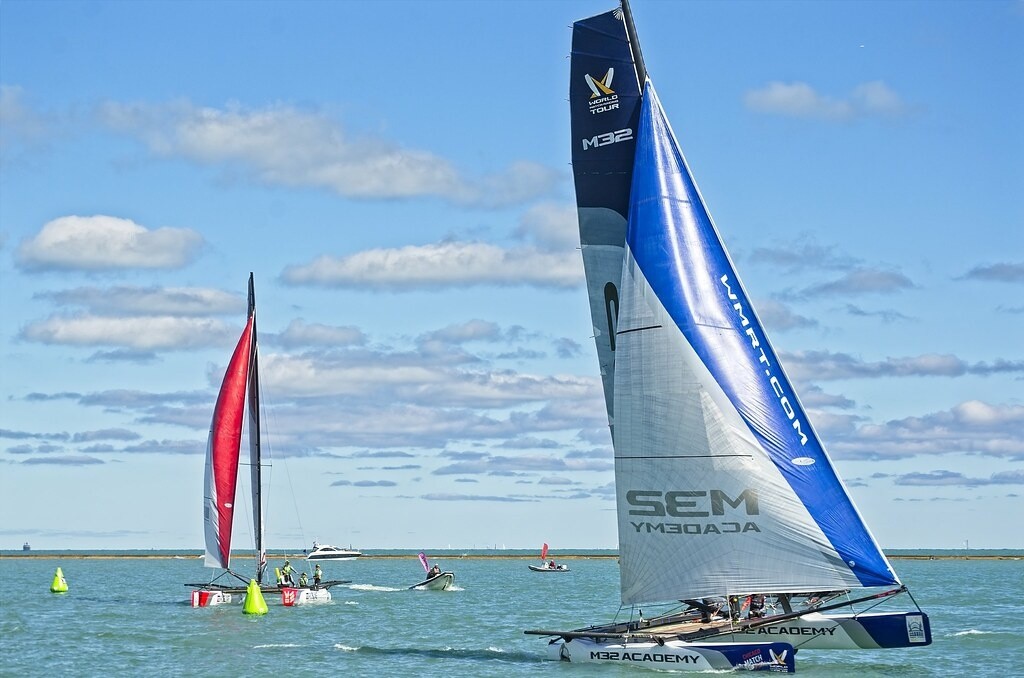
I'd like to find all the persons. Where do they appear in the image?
[282,560,323,591]
[557,564,563,570]
[548,559,555,569]
[740,594,768,620]
[426,563,442,580]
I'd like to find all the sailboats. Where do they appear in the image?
[517,5,937,675]
[180,272,353,597]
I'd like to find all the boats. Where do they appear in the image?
[303,537,362,561]
[527,562,571,572]
[409,571,455,591]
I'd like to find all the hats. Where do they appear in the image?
[302,573,307,575]
[316,564,320,567]
[285,560,290,564]
